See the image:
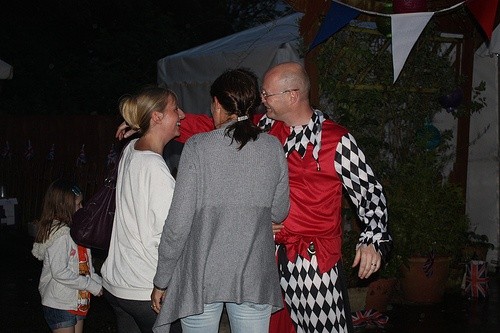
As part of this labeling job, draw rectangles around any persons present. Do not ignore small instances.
[28,180,103,333]
[150,69,291,333]
[114,61,388,333]
[97,85,185,332]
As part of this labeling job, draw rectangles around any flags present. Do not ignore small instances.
[348,307,388,330]
[459,260,489,300]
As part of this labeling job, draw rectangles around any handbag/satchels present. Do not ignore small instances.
[69,139,132,250]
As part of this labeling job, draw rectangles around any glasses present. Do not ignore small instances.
[71,184,82,197]
[260,86,300,100]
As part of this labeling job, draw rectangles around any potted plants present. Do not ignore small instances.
[399,166,472,306]
[456,231,495,261]
[365,188,411,311]
[342,229,370,312]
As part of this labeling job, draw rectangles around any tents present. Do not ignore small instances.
[157,12,307,119]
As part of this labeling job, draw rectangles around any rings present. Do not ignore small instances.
[371,263,375,266]
[152,305,155,307]
